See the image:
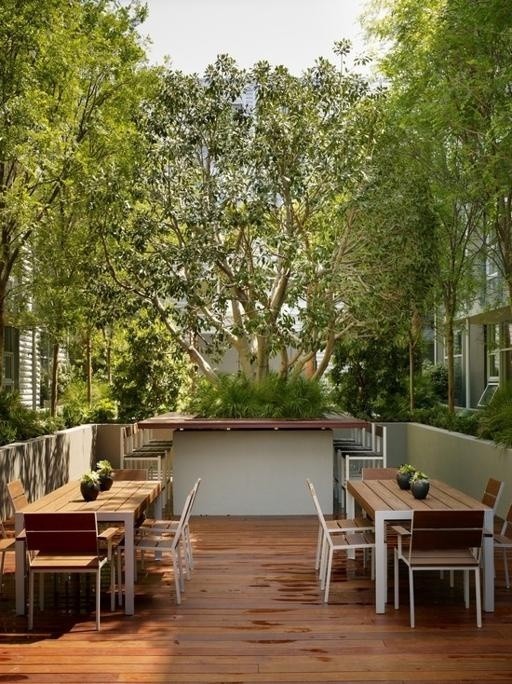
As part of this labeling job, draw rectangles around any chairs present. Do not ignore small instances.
[1,518,17,579]
[390,505,495,628]
[438,472,505,587]
[18,510,120,630]
[307,482,391,605]
[459,504,511,610]
[134,476,200,582]
[360,464,410,569]
[91,467,151,483]
[6,477,29,517]
[311,483,375,579]
[116,486,196,607]
[121,424,174,509]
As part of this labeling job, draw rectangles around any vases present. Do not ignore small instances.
[395,471,412,489]
[410,480,432,501]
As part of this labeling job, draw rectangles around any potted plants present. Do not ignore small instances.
[76,468,103,501]
[95,459,116,492]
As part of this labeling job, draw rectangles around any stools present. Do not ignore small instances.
[331,424,389,510]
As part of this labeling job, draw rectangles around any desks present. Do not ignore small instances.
[15,477,166,617]
[344,475,496,616]
